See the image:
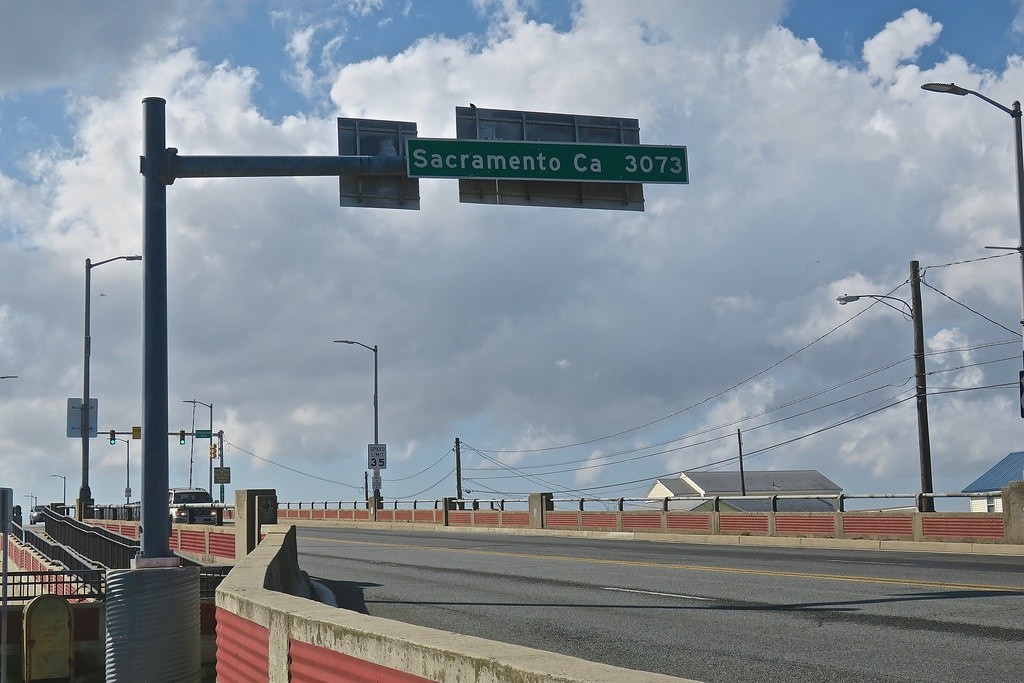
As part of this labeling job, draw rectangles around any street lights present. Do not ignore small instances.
[182,400,212,497]
[1,376,18,379]
[835,294,934,512]
[921,82,1024,418]
[79,254,142,499]
[24,495,37,506]
[333,339,378,444]
[107,437,129,503]
[49,474,66,505]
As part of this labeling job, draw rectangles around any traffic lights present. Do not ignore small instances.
[110,430,115,445]
[210,444,216,459]
[179,429,185,444]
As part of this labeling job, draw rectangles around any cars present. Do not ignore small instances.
[30,504,50,525]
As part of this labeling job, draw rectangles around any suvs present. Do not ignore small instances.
[169,487,219,525]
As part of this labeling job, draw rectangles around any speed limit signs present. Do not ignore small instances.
[368,444,386,469]
